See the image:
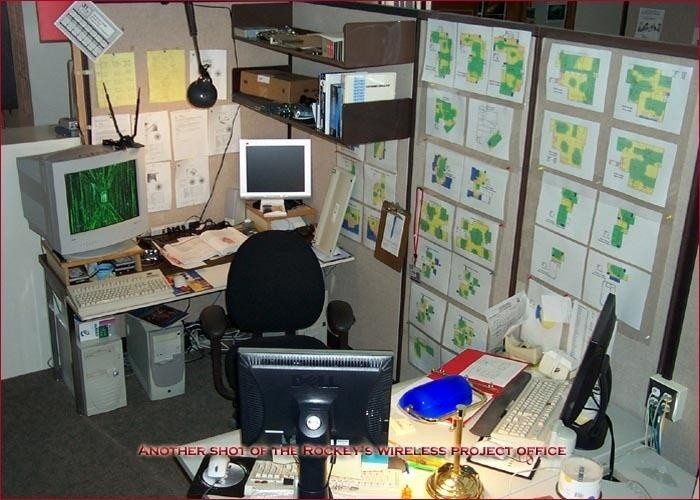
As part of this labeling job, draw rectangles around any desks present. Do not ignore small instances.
[503,443,699,499]
[33,223,356,404]
[174,340,659,500]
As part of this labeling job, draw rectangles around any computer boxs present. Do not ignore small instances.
[74,335,128,418]
[126,313,187,402]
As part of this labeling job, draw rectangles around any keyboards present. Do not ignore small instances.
[490,375,571,449]
[67,268,176,320]
[244,460,403,500]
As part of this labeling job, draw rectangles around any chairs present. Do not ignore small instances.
[191,230,353,408]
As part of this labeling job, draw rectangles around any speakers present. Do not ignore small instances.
[224,188,246,226]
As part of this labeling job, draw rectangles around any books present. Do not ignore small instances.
[163,227,249,265]
[72,313,115,343]
[317,34,397,140]
[126,304,189,329]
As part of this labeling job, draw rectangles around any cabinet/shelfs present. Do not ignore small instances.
[225,1,415,145]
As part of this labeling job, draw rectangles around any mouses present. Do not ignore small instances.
[207,454,231,479]
[480,439,509,460]
[173,274,186,287]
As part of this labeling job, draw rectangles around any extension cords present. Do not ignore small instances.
[187,335,211,350]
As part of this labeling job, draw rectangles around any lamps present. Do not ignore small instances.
[394,364,488,500]
[182,0,217,107]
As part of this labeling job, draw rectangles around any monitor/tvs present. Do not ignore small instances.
[14,143,151,260]
[234,346,395,500]
[560,292,618,452]
[238,138,313,218]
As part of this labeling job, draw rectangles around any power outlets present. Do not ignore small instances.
[645,372,687,423]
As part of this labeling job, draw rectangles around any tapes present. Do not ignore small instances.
[519,341,531,348]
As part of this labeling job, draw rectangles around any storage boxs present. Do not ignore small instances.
[239,67,318,104]
